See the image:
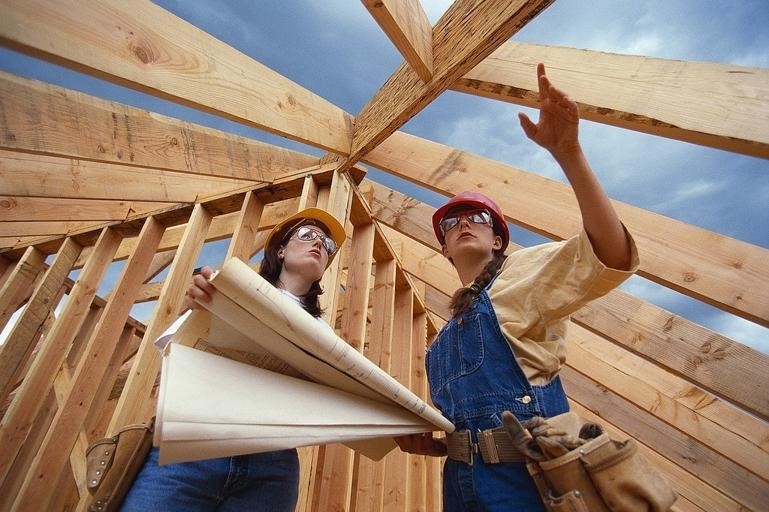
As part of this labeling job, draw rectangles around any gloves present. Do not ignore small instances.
[502,409,603,459]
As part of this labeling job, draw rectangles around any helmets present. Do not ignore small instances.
[433,192,509,253]
[266,208,347,269]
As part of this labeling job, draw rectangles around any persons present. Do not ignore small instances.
[392,63,641,512]
[119,207,347,512]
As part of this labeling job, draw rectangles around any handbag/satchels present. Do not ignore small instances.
[85,423,152,511]
[527,432,678,511]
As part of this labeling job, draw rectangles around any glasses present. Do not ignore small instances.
[440,210,492,230]
[290,227,337,255]
